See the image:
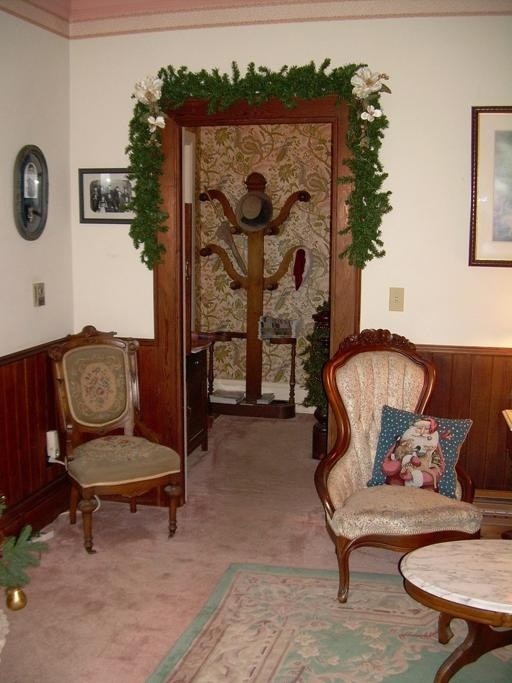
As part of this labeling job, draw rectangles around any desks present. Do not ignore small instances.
[391,535,510,682]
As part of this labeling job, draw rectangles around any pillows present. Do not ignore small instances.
[364,399,473,501]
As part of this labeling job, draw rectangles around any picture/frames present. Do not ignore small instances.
[76,166,139,225]
[465,103,511,267]
[14,143,52,242]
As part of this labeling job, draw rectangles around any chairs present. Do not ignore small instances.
[43,324,185,553]
[314,326,488,605]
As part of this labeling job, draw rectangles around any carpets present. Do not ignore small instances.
[141,560,509,682]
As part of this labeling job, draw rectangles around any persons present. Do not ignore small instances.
[27,164,37,197]
[89,181,132,211]
